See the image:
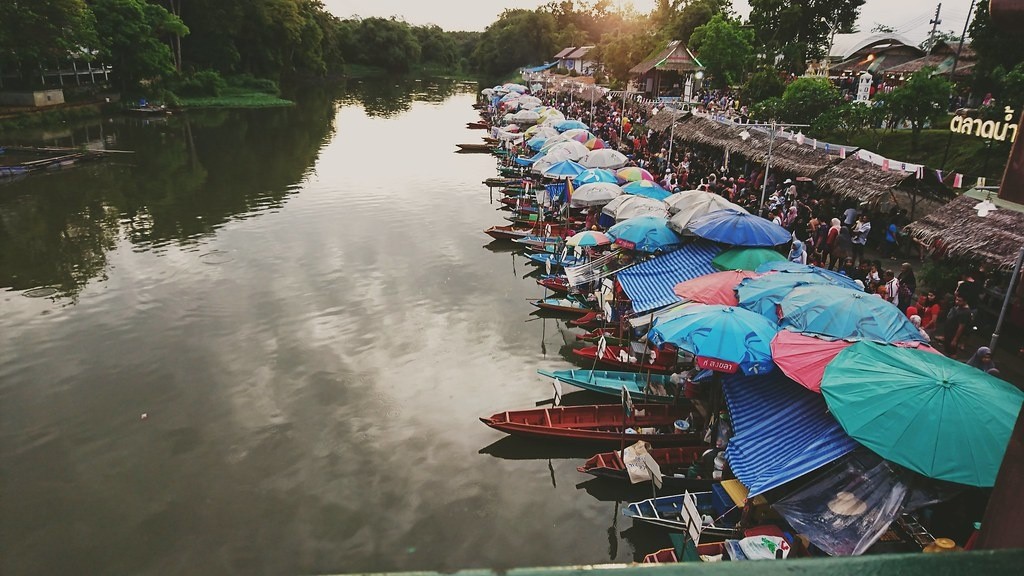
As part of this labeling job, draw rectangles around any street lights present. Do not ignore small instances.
[820,16,839,75]
[737,121,811,217]
[606,91,645,144]
[649,101,698,169]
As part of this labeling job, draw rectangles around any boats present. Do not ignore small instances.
[478,403,709,444]
[530,298,589,313]
[567,311,603,326]
[622,478,783,534]
[643,534,791,563]
[572,346,689,373]
[503,216,585,229]
[457,101,583,224]
[574,327,631,346]
[576,445,726,483]
[525,244,576,254]
[484,226,571,240]
[510,234,564,244]
[0,149,105,178]
[127,99,166,118]
[537,370,686,402]
[523,252,589,267]
[536,278,572,293]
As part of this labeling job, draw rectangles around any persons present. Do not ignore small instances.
[518,53,1008,375]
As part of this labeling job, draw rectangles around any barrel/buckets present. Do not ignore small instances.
[674,420,690,435]
[529,214,538,220]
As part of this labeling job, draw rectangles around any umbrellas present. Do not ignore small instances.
[481,83,1023,488]
